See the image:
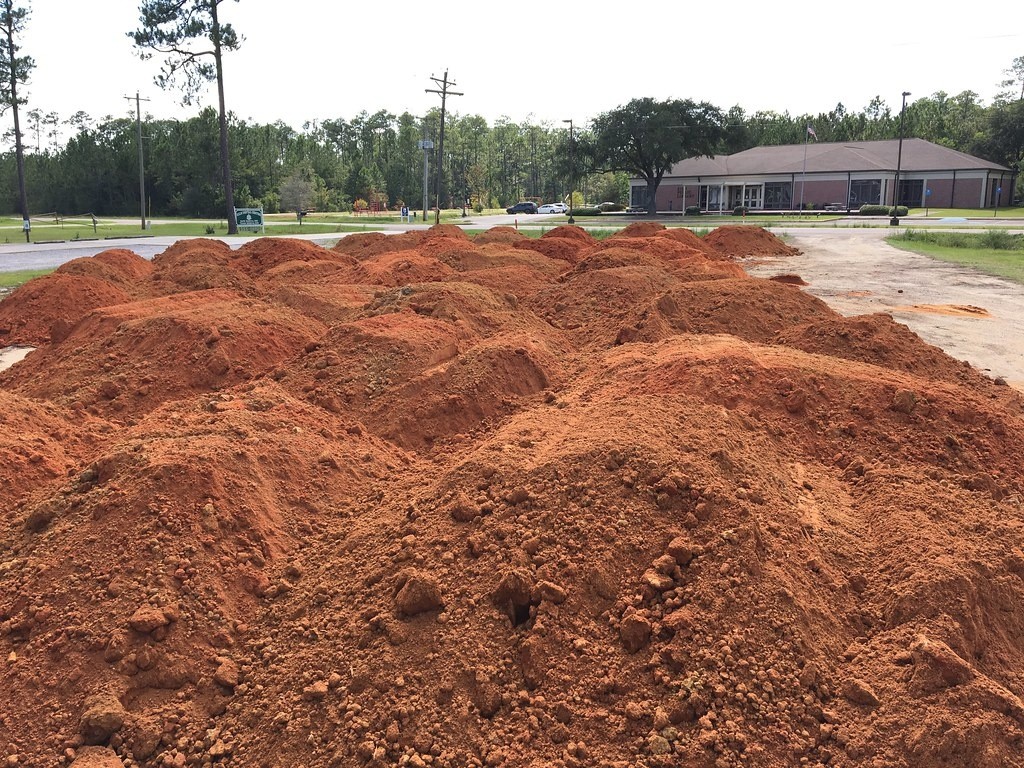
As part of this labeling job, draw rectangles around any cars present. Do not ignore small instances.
[553,202,569,213]
[536,203,563,214]
[594,201,615,212]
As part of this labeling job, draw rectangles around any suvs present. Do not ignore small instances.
[506,202,538,215]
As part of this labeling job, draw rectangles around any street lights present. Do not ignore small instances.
[890,91,912,226]
[562,118,575,224]
